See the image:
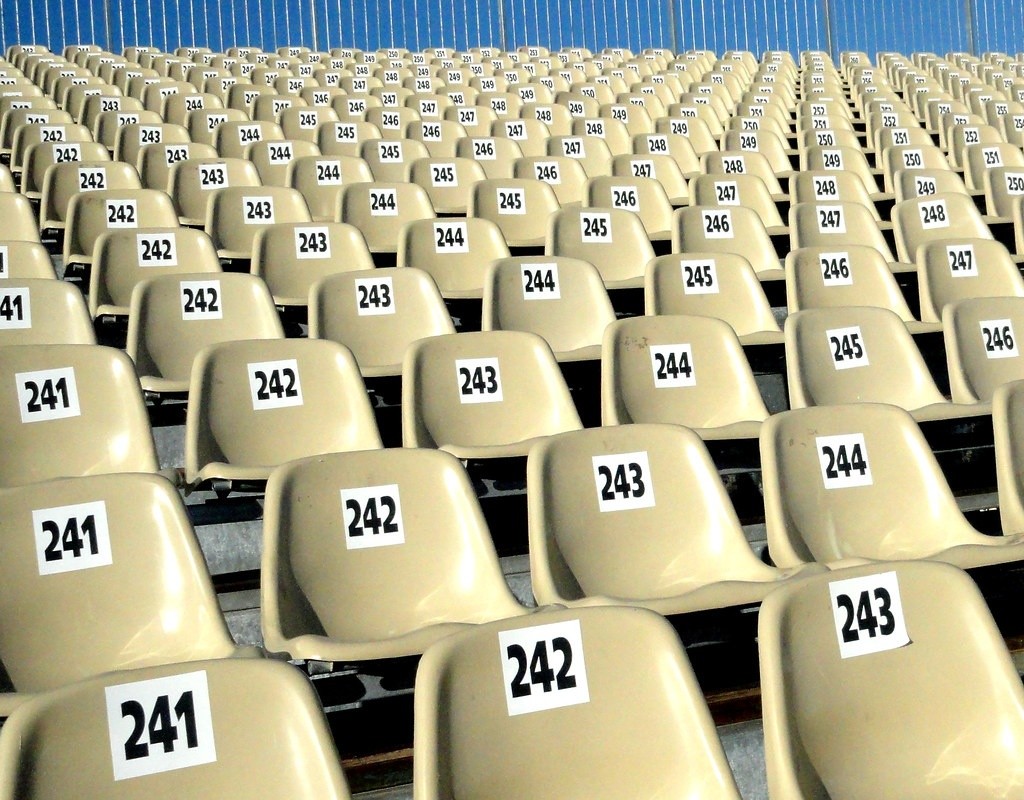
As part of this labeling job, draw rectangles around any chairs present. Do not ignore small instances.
[0,44,1024,800]
